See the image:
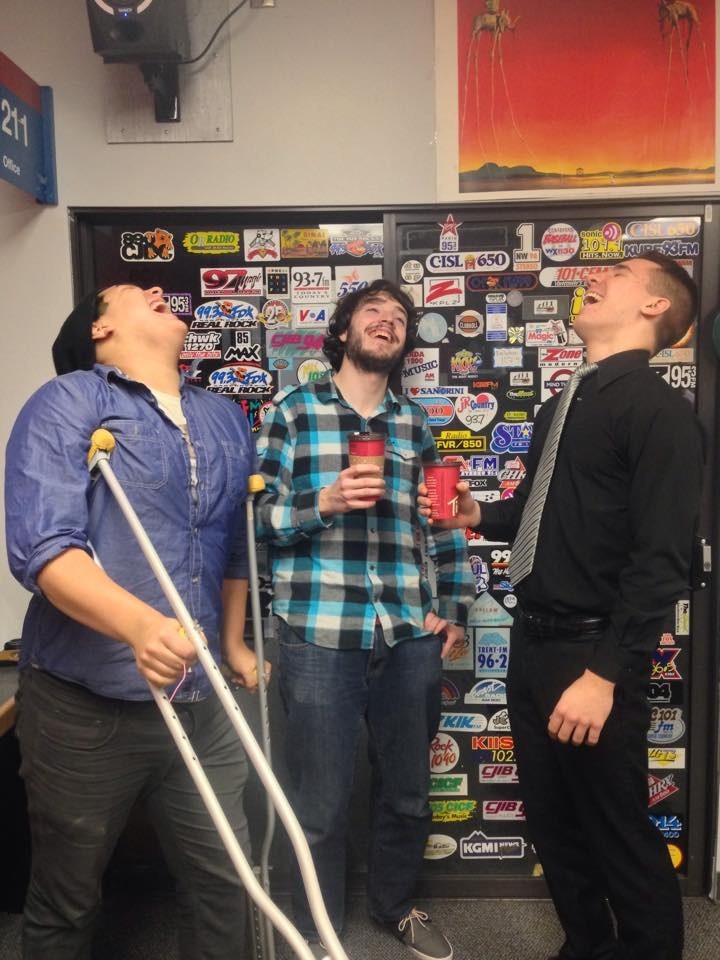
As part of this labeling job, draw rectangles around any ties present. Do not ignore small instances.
[509,360,600,585]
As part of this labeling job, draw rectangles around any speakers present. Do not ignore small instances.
[87,0,189,62]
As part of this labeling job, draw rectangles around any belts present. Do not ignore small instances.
[518,610,608,633]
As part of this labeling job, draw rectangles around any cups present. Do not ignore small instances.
[346,431,388,501]
[421,462,460,520]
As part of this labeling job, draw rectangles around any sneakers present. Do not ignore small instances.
[374,905,453,960]
[292,934,333,960]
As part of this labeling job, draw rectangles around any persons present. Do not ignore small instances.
[418,249,712,960]
[253,280,469,960]
[5,283,273,960]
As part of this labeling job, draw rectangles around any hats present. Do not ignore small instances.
[52,290,97,374]
